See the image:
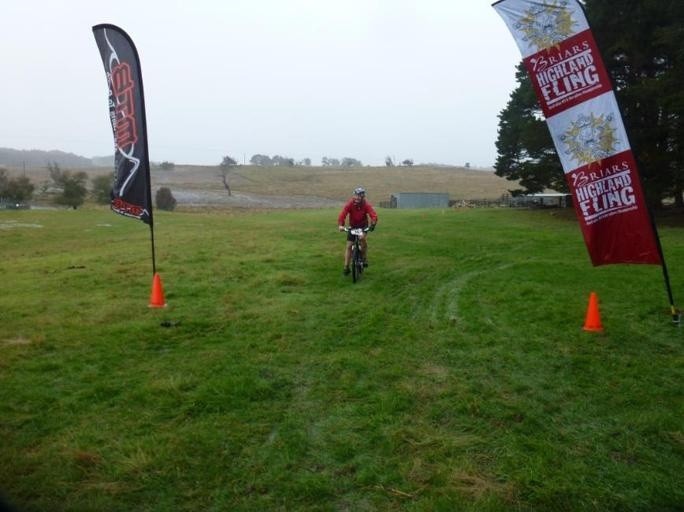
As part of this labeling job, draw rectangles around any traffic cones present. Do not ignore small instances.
[582,291,603,333]
[148,272,165,309]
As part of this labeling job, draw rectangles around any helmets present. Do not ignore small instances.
[353,187,366,196]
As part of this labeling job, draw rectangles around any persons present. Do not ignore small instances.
[338,187,377,276]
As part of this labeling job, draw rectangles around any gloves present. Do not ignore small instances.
[369,224,376,232]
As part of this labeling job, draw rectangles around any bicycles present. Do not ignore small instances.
[342,226,368,284]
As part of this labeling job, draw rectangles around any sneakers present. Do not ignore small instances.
[363,258,368,264]
[343,265,349,272]
